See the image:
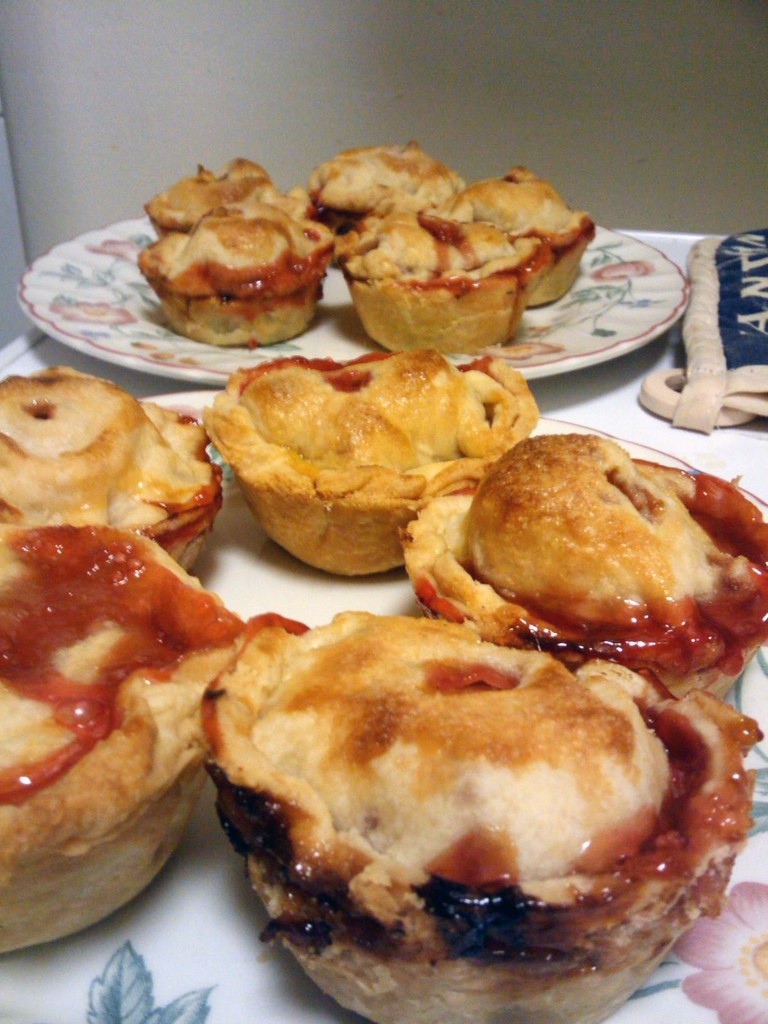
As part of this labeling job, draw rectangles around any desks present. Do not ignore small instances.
[0,228,768,1024]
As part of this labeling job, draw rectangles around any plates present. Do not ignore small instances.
[0,392,768,1023]
[19,214,687,387]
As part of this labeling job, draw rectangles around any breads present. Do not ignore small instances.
[0,144,768,1024]
